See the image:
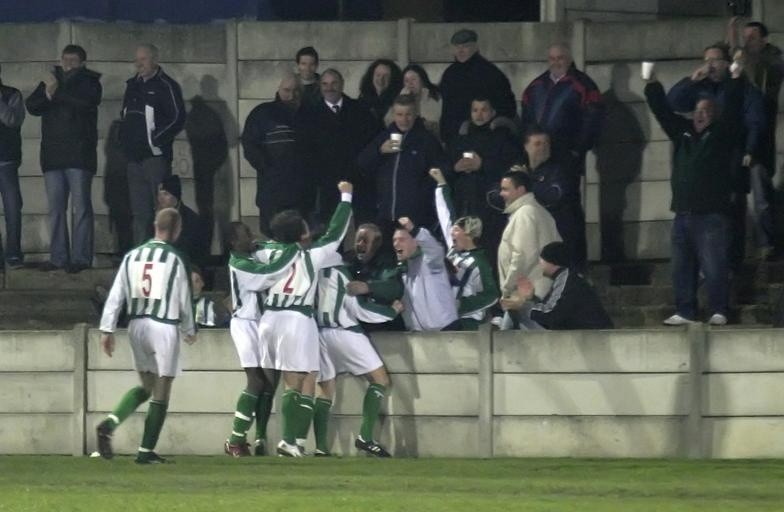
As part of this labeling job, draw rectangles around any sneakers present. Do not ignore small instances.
[135,452,164,463]
[97,424,111,456]
[356,434,390,456]
[709,314,727,325]
[664,314,702,325]
[225,438,327,457]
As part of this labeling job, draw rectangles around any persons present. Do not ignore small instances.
[26,45,102,273]
[0,78,25,276]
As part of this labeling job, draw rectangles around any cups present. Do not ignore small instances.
[729,60,742,71]
[389,133,401,151]
[463,152,471,158]
[641,62,654,80]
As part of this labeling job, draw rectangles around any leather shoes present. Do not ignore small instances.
[40,261,87,272]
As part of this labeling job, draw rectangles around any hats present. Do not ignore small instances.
[451,30,478,44]
[541,241,571,266]
[158,175,181,200]
